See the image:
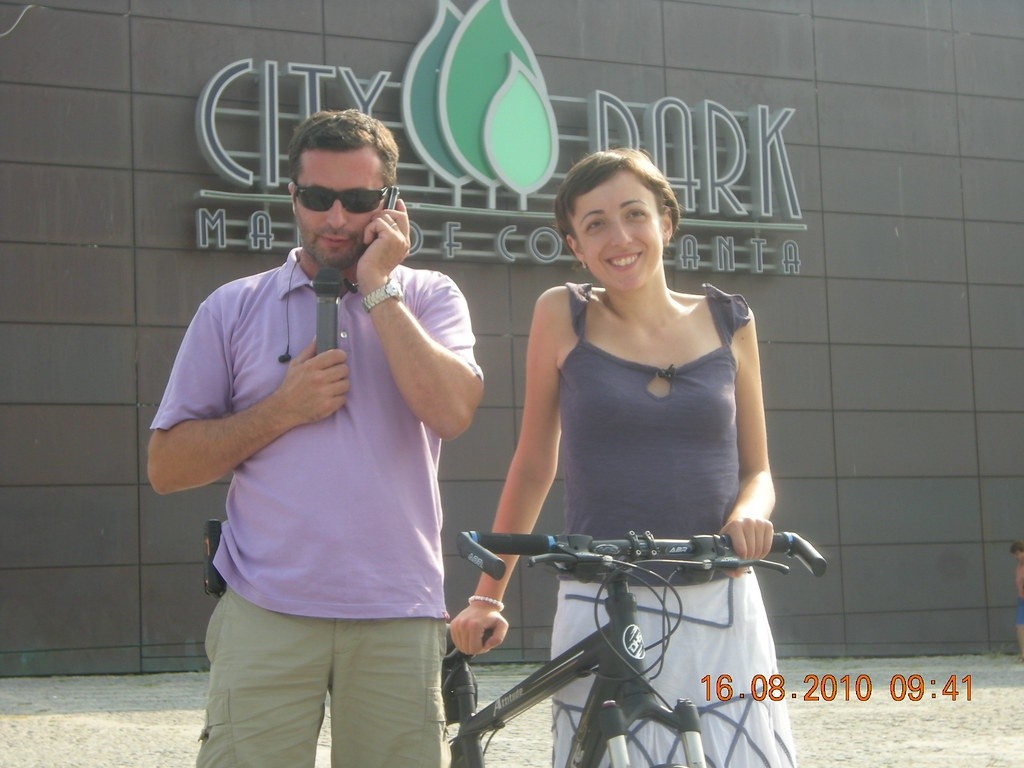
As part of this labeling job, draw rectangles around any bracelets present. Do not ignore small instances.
[390,222,398,227]
[467,594,505,613]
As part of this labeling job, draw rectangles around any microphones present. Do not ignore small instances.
[312,267,344,356]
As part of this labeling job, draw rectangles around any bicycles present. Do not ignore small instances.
[201,519,828,768]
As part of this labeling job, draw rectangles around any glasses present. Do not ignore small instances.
[294,182,388,213]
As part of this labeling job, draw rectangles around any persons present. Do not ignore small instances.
[145,106,486,768]
[448,144,800,768]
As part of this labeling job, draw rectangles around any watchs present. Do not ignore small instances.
[360,276,404,314]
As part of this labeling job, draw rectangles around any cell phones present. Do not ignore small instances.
[387,186,400,211]
